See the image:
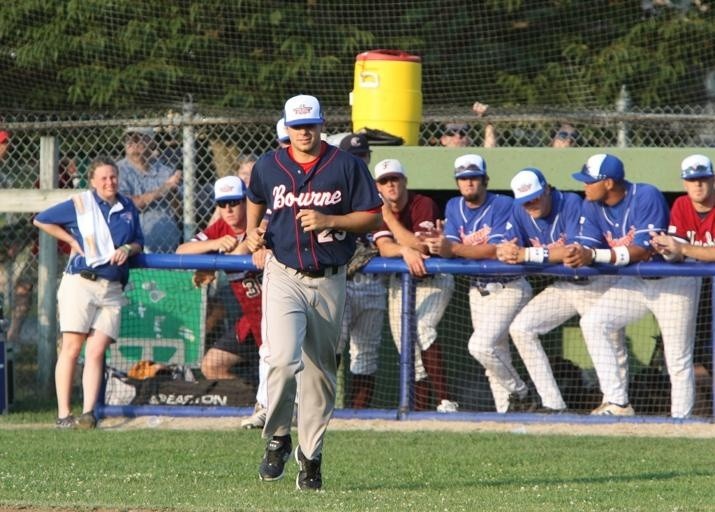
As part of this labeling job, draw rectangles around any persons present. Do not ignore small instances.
[246,93,384,493]
[1,123,303,430]
[319,122,715,415]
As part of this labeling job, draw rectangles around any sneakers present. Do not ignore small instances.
[294,443,322,493]
[240,408,270,430]
[79,410,99,432]
[435,398,459,413]
[258,435,293,482]
[505,389,539,414]
[54,416,78,431]
[589,402,636,416]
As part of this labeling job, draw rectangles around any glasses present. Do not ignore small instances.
[555,130,582,141]
[442,127,471,137]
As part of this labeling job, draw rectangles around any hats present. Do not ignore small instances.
[283,94,323,127]
[374,158,406,182]
[510,167,547,206]
[454,153,487,179]
[214,174,247,202]
[572,153,625,184]
[119,120,154,147]
[680,153,714,180]
[276,117,290,142]
[339,133,373,154]
